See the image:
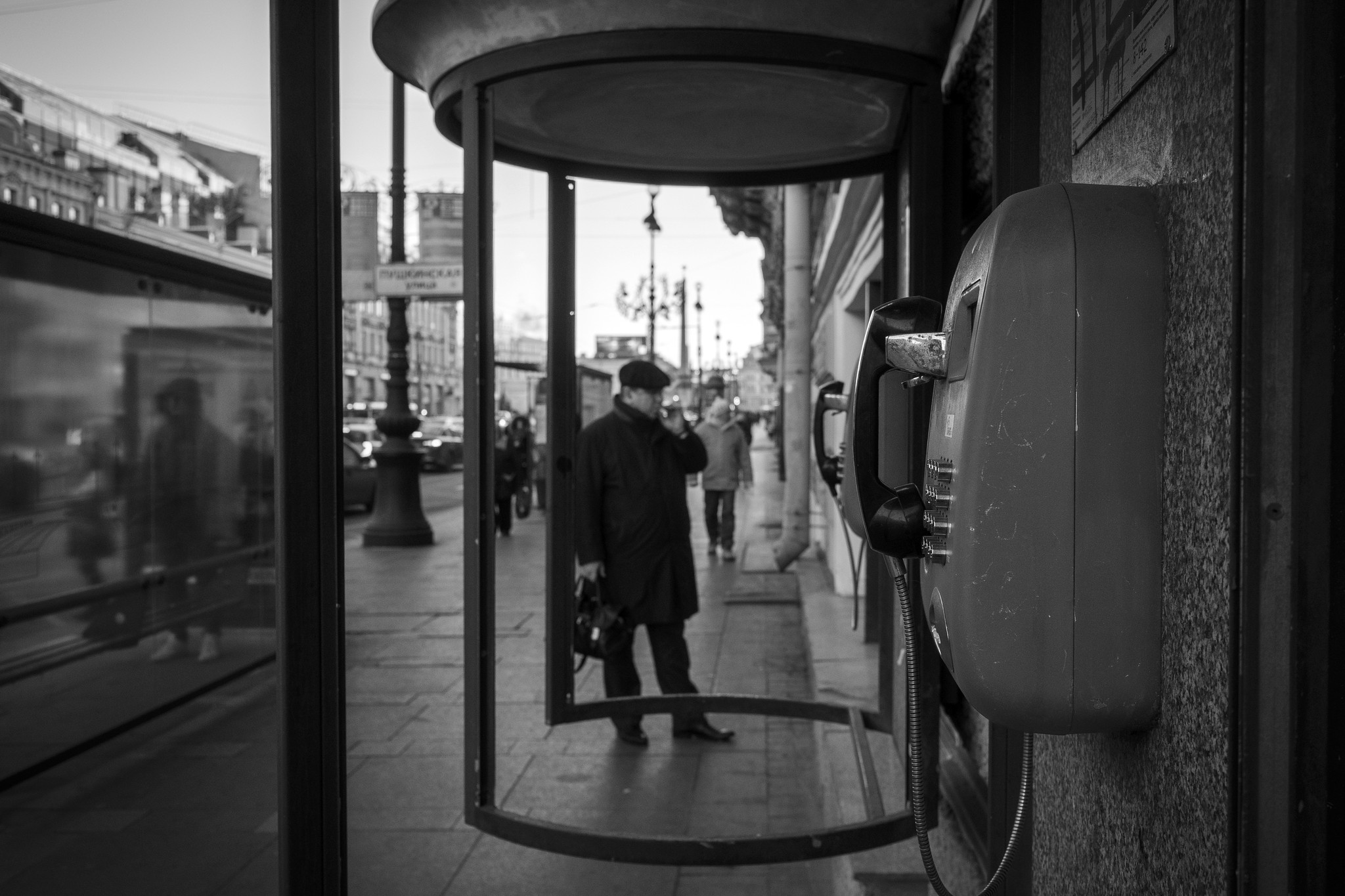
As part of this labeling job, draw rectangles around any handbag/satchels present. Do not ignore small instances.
[574,576,624,673]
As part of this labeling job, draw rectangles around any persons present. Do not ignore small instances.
[150,616,226,665]
[490,408,544,544]
[685,397,755,562]
[572,361,739,742]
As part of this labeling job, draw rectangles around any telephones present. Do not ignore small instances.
[813,380,845,486]
[842,184,1168,736]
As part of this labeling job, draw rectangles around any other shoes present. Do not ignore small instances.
[709,541,717,555]
[723,549,735,561]
[148,634,188,663]
[199,632,222,663]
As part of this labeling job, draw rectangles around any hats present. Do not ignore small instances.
[620,360,670,388]
[711,396,731,416]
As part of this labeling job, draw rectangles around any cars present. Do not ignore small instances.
[161,390,500,547]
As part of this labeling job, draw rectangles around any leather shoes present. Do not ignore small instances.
[672,717,735,741]
[616,725,649,747]
